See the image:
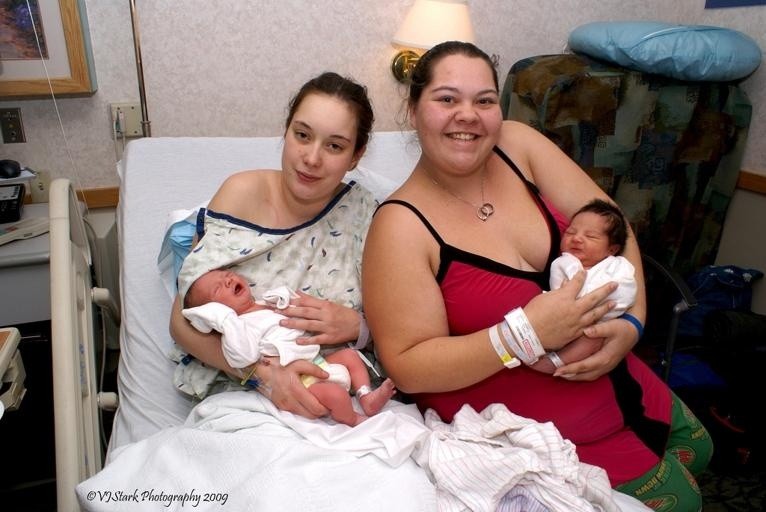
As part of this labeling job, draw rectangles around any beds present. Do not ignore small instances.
[48,130,653,512]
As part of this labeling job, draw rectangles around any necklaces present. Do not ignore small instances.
[418,154,496,221]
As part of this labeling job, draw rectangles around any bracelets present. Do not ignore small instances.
[619,311,644,339]
[347,311,372,351]
[489,307,544,370]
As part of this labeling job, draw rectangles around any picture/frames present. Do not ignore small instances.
[0,0,98,101]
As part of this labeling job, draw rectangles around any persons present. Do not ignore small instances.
[169,72,385,403]
[528,199,638,374]
[184,268,397,427]
[361,42,714,512]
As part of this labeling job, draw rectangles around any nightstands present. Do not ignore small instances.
[0,201,85,512]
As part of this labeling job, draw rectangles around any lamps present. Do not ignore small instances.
[391,0,474,83]
